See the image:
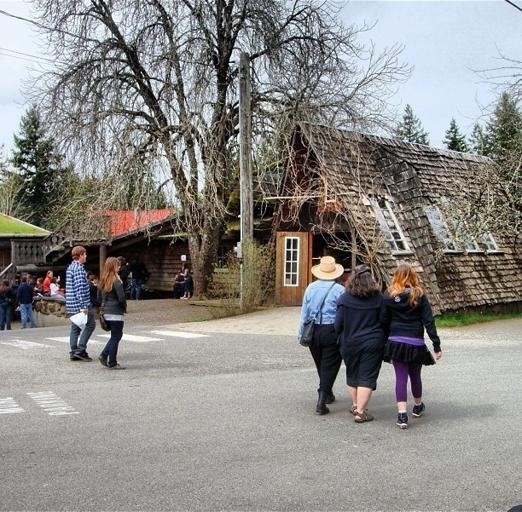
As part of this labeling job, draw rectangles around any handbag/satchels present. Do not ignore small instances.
[300,320,315,346]
[99,314,111,331]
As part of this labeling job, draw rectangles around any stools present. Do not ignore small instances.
[27,291,66,316]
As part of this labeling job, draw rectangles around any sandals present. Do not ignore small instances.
[349,406,373,423]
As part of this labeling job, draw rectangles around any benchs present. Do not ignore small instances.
[144,268,182,292]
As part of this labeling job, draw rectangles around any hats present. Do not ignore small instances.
[311,256,345,280]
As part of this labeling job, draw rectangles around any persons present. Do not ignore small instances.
[294,255,346,416]
[95,256,129,371]
[174,262,192,302]
[378,261,441,429]
[65,247,97,364]
[1,256,142,332]
[334,263,385,423]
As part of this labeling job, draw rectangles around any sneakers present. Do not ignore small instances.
[109,364,126,370]
[98,355,108,367]
[396,413,408,429]
[412,403,426,417]
[70,352,91,362]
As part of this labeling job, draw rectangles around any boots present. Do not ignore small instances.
[316,391,335,415]
